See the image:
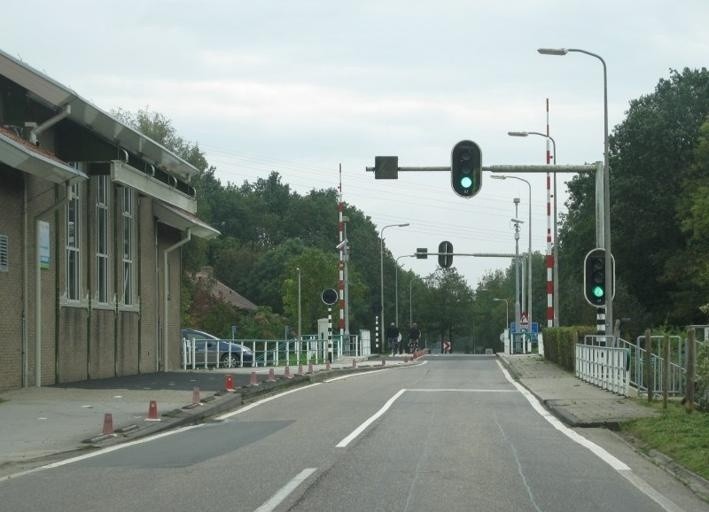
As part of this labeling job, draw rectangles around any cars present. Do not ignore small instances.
[182,328,254,369]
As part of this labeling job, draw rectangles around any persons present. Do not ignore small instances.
[408,323,422,353]
[387,321,399,353]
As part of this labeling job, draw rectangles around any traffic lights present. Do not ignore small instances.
[451,140,482,198]
[584,248,616,307]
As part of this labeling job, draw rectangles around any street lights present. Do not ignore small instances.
[491,131,560,332]
[296,265,301,335]
[537,47,613,346]
[381,223,429,355]
